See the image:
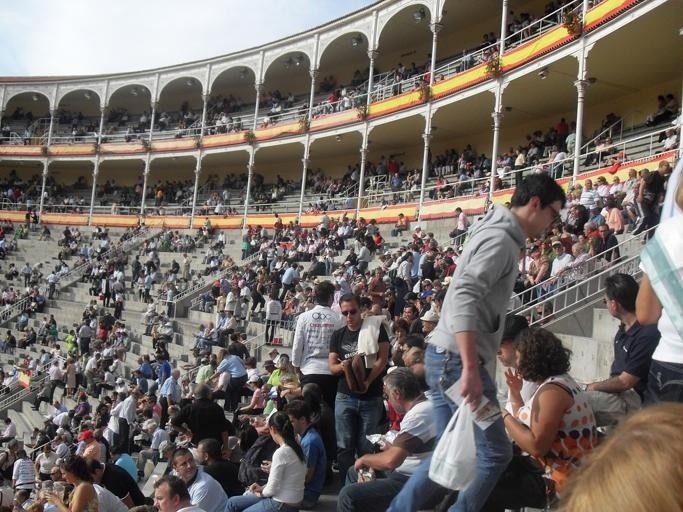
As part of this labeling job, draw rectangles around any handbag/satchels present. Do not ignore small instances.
[238,435,276,486]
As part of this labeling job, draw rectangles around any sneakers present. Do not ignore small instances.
[31,406,39,411]
[138,471,144,477]
[88,392,99,398]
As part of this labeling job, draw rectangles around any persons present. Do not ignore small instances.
[1,1,683,511]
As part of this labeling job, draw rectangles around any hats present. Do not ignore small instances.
[414,226,420,231]
[80,431,92,439]
[116,378,123,384]
[159,440,173,458]
[420,311,439,322]
[502,314,528,340]
[246,375,259,385]
[56,428,64,434]
[263,359,276,366]
[552,241,562,247]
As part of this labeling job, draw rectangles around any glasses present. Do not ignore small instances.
[342,309,359,316]
[547,205,562,229]
